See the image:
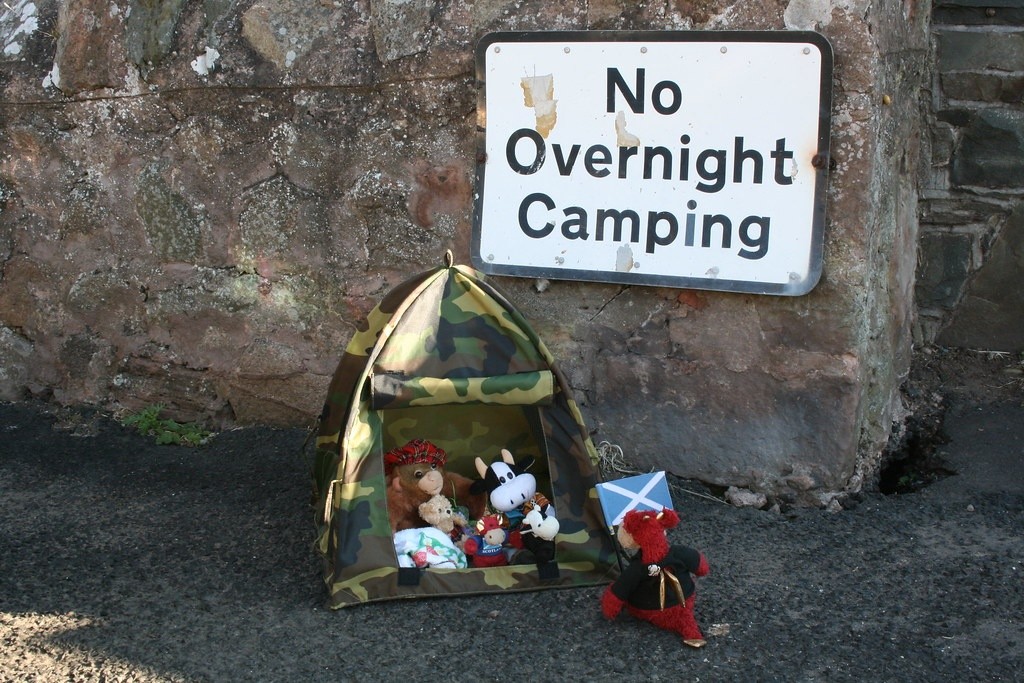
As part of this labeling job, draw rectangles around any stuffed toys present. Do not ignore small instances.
[602,509,708,641]
[383,437,560,569]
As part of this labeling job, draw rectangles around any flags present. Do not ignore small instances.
[596,472,674,526]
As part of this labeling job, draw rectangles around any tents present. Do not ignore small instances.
[312,249,622,611]
